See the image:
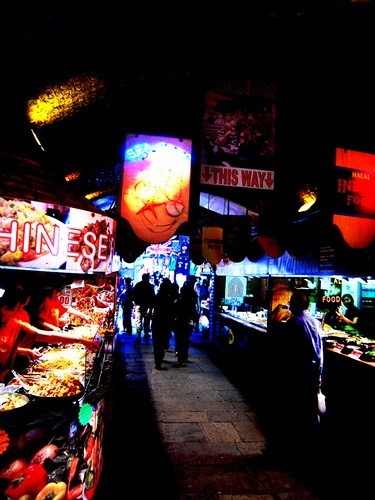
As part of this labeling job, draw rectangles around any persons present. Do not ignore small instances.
[0,275,102,371]
[258,288,324,473]
[174,285,201,367]
[333,294,361,336]
[122,272,209,338]
[148,283,175,370]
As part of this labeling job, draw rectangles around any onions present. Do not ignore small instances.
[0,444,58,481]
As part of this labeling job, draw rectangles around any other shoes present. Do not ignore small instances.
[144,334,151,339]
[155,365,168,370]
[175,361,187,368]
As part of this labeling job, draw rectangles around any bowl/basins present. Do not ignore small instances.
[0,378,85,423]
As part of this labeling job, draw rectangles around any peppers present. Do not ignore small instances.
[5,465,67,500]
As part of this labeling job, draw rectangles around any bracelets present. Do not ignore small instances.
[76,337,80,343]
